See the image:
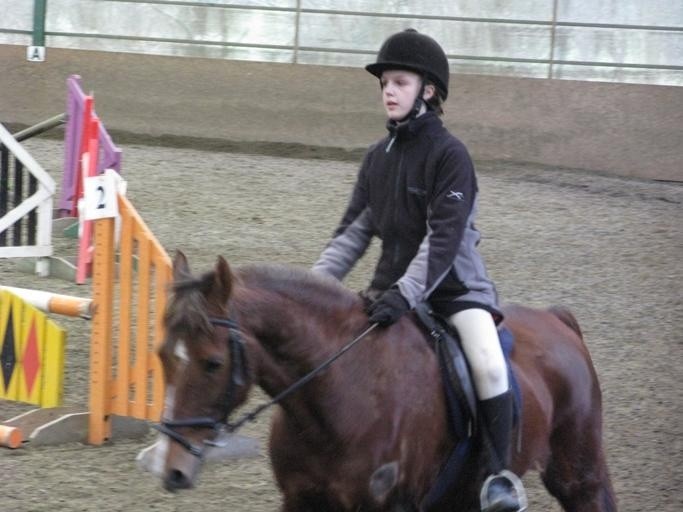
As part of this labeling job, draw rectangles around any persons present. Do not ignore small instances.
[309,28,521,512]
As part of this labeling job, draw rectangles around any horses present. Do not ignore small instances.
[147,247,622,512]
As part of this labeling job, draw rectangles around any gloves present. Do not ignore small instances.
[366,288,410,329]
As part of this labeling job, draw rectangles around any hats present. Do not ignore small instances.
[364,27,450,102]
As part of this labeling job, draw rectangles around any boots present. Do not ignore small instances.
[478,384,518,511]
